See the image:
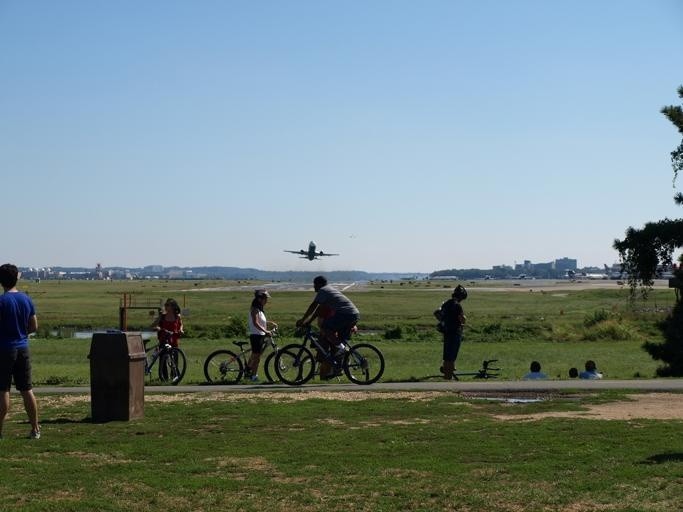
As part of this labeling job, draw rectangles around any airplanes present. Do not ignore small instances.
[283,241,340,260]
[511,273,533,280]
[565,270,609,281]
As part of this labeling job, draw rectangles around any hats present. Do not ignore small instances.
[255,289,271,298]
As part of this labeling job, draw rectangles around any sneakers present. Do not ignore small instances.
[30,428,41,440]
[334,343,346,357]
[243,369,259,382]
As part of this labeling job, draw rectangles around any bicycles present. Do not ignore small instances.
[202,322,303,386]
[274,320,385,385]
[422,357,500,381]
[141,328,187,385]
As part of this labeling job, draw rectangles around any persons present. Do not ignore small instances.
[0,268,39,438]
[316,305,357,381]
[433,284,467,379]
[245,289,278,381]
[579,360,601,378]
[568,367,579,378]
[522,361,547,380]
[296,275,360,378]
[150,297,182,382]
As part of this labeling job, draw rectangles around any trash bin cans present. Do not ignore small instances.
[87,329,146,422]
